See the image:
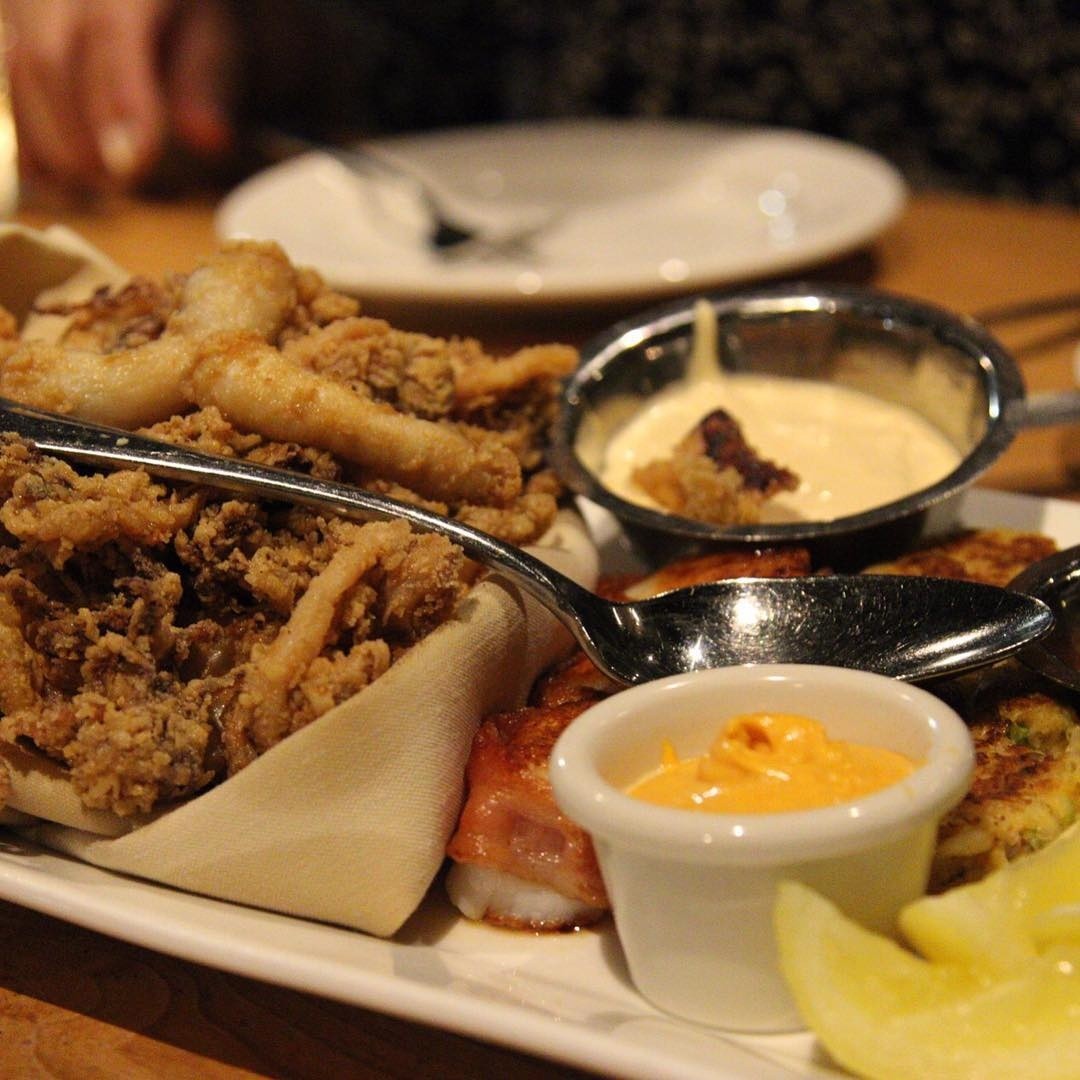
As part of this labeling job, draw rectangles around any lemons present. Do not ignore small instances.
[773,808,1080,1079]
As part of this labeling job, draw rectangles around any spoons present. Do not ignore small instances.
[1,399,1053,683]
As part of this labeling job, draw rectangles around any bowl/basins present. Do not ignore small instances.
[548,288,1029,574]
[547,662,974,1035]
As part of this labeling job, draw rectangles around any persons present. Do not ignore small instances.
[10,3,1080,216]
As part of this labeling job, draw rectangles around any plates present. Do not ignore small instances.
[214,124,914,329]
[1,484,1080,1080]
